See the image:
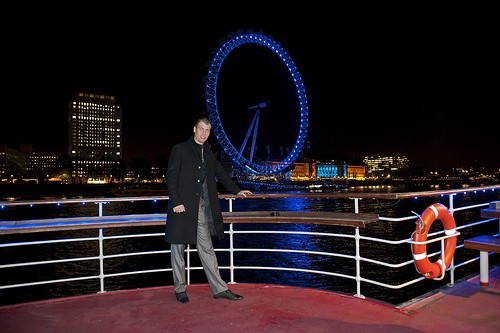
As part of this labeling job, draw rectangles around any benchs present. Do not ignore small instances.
[464,235,500,286]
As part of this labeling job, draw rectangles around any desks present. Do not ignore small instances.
[481,209,500,218]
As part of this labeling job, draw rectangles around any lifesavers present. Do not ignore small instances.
[411,203,457,277]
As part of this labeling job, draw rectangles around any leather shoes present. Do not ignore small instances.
[213,288,243,299]
[175,291,189,303]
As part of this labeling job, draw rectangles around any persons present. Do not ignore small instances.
[165,118,254,304]
